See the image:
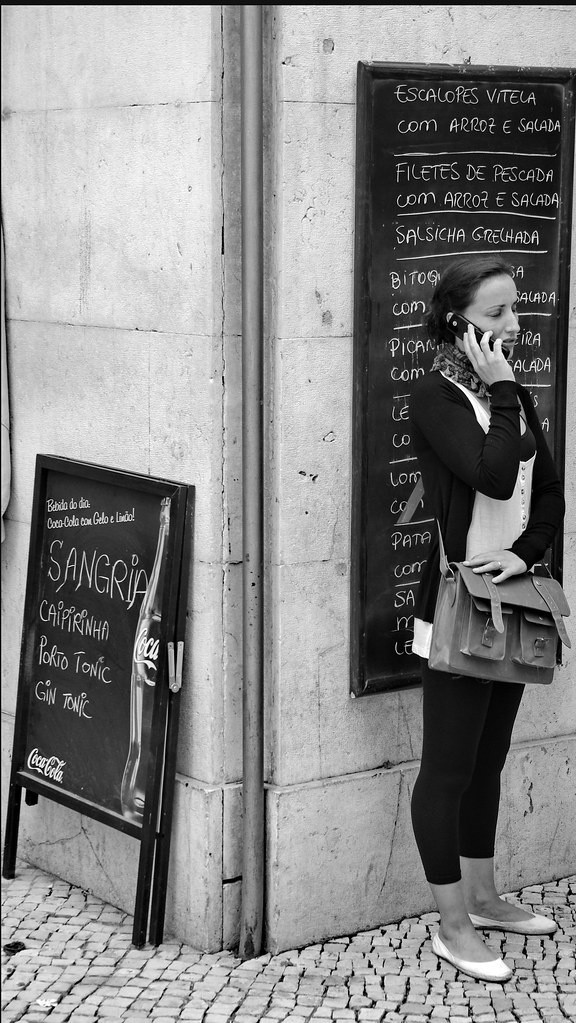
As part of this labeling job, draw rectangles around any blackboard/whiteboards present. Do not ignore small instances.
[14,454,196,841]
[349,60,576,697]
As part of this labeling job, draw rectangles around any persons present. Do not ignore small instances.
[406,257,567,982]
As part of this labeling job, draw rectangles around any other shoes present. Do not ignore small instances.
[431,932,512,981]
[468,904,557,934]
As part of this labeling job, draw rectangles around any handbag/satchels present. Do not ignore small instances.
[428,559,572,684]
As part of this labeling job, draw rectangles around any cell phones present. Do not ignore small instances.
[448,312,510,361]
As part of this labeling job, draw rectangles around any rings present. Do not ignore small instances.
[498,562,502,568]
